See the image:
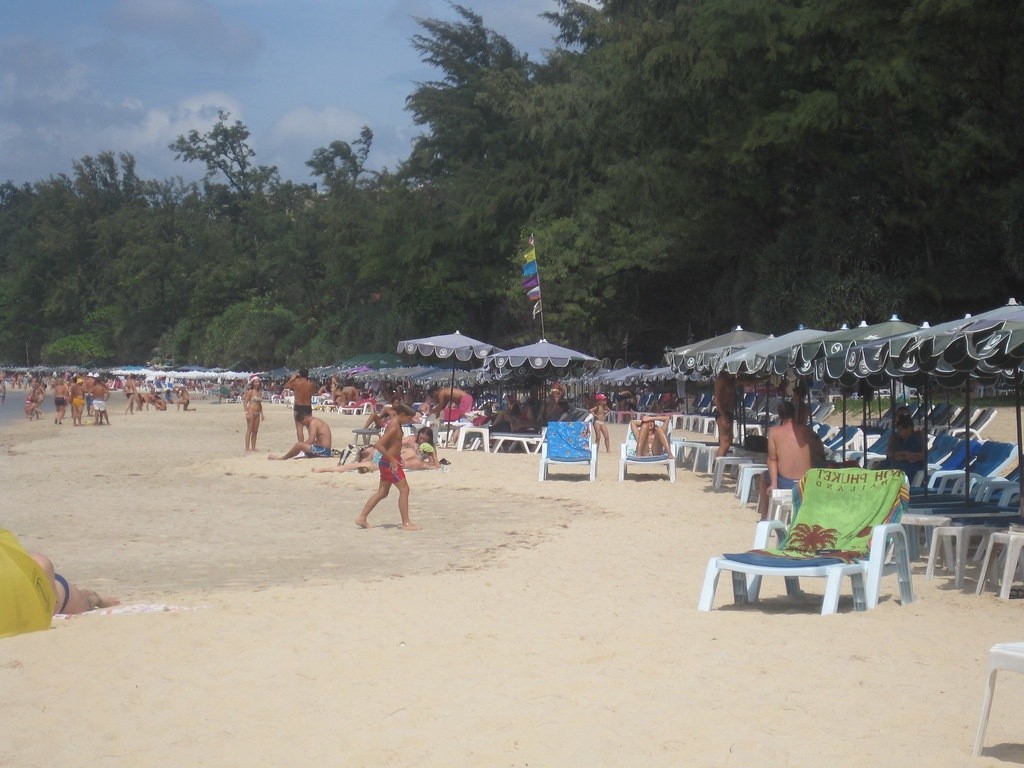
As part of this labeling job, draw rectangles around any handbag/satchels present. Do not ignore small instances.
[340,444,361,466]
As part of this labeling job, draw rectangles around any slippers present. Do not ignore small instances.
[440,458,451,465]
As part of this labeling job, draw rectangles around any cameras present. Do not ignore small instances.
[296,375,299,378]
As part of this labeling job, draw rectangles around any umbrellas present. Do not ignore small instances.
[0,297,1024,498]
[0,528,57,638]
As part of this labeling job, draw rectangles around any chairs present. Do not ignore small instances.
[130,375,1024,757]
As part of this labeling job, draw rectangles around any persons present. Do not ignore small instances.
[30,553,121,614]
[792,388,809,424]
[895,407,909,419]
[874,416,923,483]
[810,428,826,464]
[0,368,675,532]
[715,365,737,457]
[759,402,816,520]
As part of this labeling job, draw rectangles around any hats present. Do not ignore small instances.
[596,393,608,401]
[419,443,434,457]
